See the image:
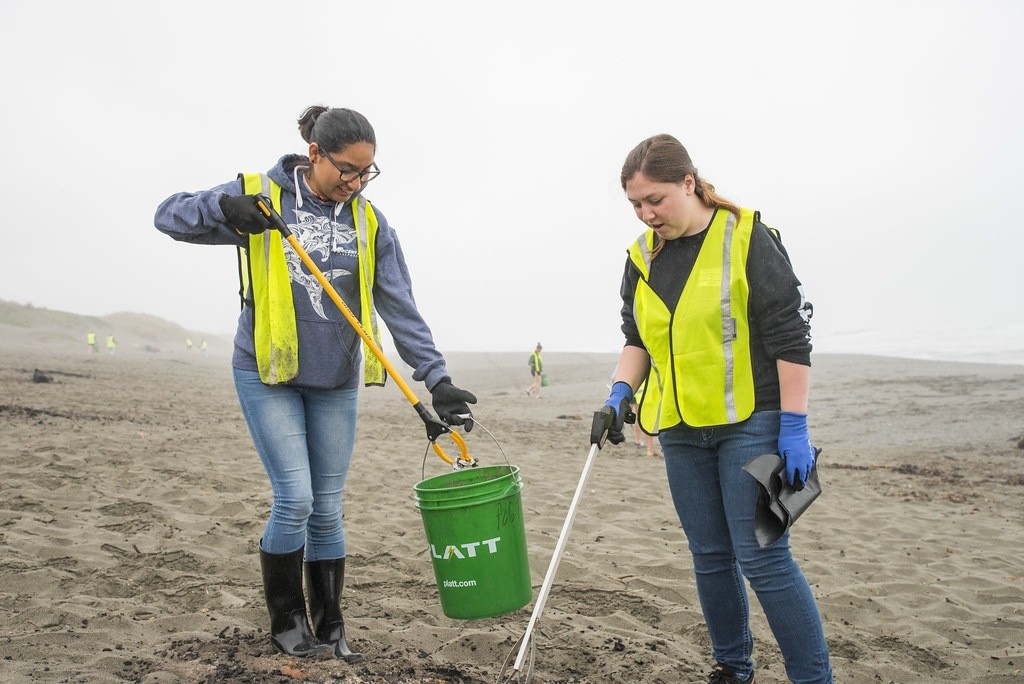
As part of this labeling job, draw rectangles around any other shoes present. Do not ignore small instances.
[707,663,755,684]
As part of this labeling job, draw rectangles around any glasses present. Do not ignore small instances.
[320,144,380,182]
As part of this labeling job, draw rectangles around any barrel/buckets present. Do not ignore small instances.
[413,413,532,620]
[541,374,549,387]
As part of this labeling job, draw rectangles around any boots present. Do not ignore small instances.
[303,557,363,664]
[259,537,329,658]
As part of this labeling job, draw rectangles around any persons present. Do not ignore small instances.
[602,135,834,684]
[185,337,192,351]
[527,341,544,399]
[107,333,118,354]
[154,102,476,664]
[86,329,96,355]
[198,338,207,349]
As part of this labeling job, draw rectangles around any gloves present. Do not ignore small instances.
[219,193,274,235]
[778,412,816,492]
[599,381,634,445]
[430,376,477,432]
